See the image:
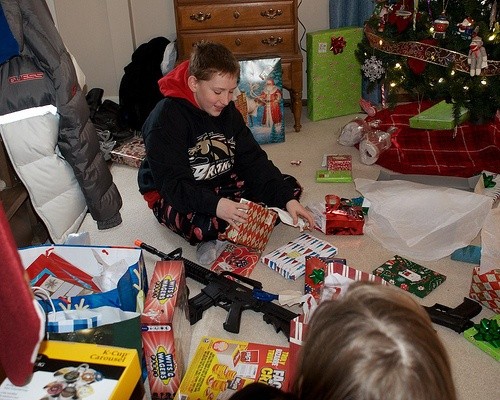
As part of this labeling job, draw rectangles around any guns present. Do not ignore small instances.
[420,297,483,334]
[134,240,301,342]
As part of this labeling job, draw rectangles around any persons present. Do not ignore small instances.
[137,42,314,245]
[229,279,458,400]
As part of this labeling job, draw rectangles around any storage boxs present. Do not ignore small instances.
[373,255,447,298]
[307,26,363,121]
[234,55,285,144]
[173,336,289,400]
[303,203,365,236]
[263,233,338,279]
[108,133,147,167]
[0,340,149,400]
[315,170,353,182]
[470,267,500,314]
[140,261,192,400]
[327,155,352,170]
[228,199,278,252]
[411,100,468,129]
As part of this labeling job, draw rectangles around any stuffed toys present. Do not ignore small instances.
[467,35,488,77]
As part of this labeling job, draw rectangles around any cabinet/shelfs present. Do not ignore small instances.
[174,0,303,133]
